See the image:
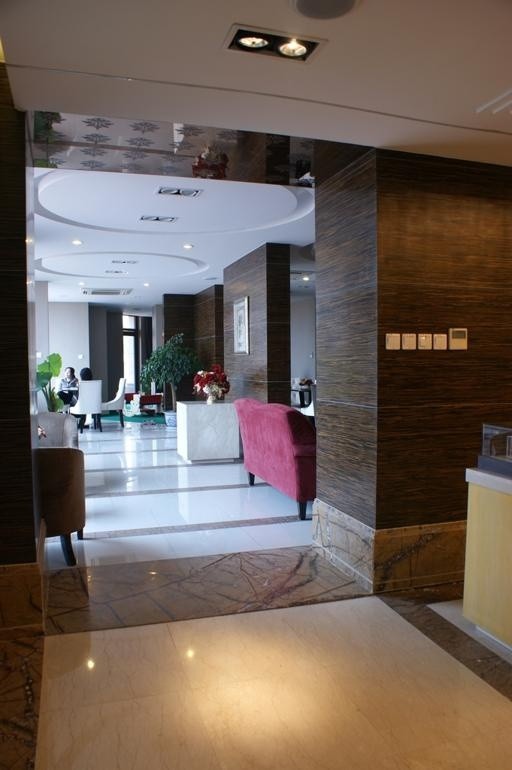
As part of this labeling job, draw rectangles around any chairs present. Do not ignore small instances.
[35,410,87,567]
[70,375,128,433]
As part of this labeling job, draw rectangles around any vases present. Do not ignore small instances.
[206,395,216,404]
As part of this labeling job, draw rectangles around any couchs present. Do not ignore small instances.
[234,396,315,521]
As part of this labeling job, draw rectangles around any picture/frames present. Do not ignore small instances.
[232,295,250,356]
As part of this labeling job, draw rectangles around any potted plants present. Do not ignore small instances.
[137,331,206,427]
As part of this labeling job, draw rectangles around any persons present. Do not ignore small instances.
[59,367,78,398]
[56,367,93,408]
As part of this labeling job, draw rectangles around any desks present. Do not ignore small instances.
[291,387,311,406]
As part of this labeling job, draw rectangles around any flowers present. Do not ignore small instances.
[191,362,231,400]
